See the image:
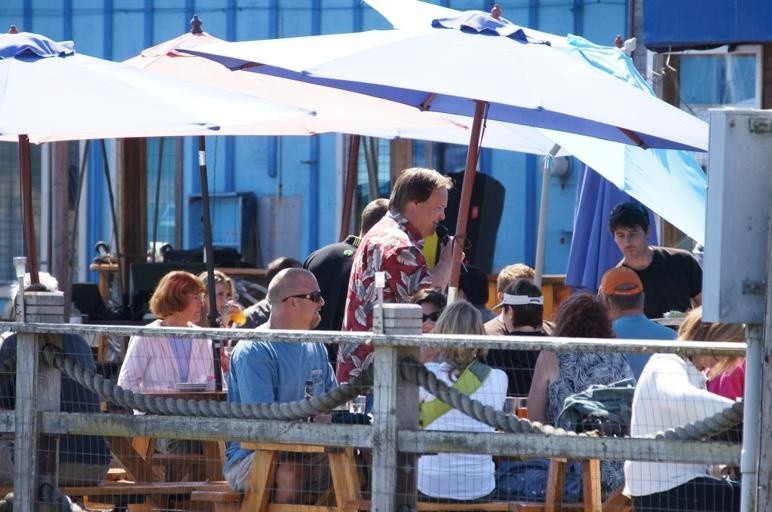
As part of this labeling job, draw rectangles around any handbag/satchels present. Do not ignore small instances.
[555,378,635,435]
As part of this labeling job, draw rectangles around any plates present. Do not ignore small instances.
[175,382,207,394]
[650,317,685,329]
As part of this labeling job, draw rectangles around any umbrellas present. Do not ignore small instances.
[0,22,319,285]
[173,6,718,286]
[110,18,473,391]
[377,105,564,166]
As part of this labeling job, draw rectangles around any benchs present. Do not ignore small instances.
[1,475,238,511]
[188,484,243,511]
[340,491,641,511]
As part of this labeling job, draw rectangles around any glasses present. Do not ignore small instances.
[422,312,440,322]
[281,291,323,303]
[610,202,646,215]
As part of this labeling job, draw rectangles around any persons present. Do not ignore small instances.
[238,254,306,327]
[299,196,393,379]
[194,270,242,328]
[405,286,449,361]
[595,198,706,321]
[337,166,468,413]
[404,298,512,505]
[455,265,501,321]
[226,264,352,508]
[1,284,112,505]
[480,277,565,400]
[624,304,752,511]
[591,268,687,389]
[114,267,228,485]
[476,258,561,340]
[521,291,640,494]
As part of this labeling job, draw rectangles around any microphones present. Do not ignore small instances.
[436,225,469,277]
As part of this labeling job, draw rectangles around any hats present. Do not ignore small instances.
[598,266,644,297]
[493,292,544,310]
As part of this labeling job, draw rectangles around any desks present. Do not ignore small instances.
[236,436,373,512]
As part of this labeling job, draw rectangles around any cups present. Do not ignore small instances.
[348,395,367,415]
[516,397,529,419]
[503,396,517,417]
[222,298,246,327]
